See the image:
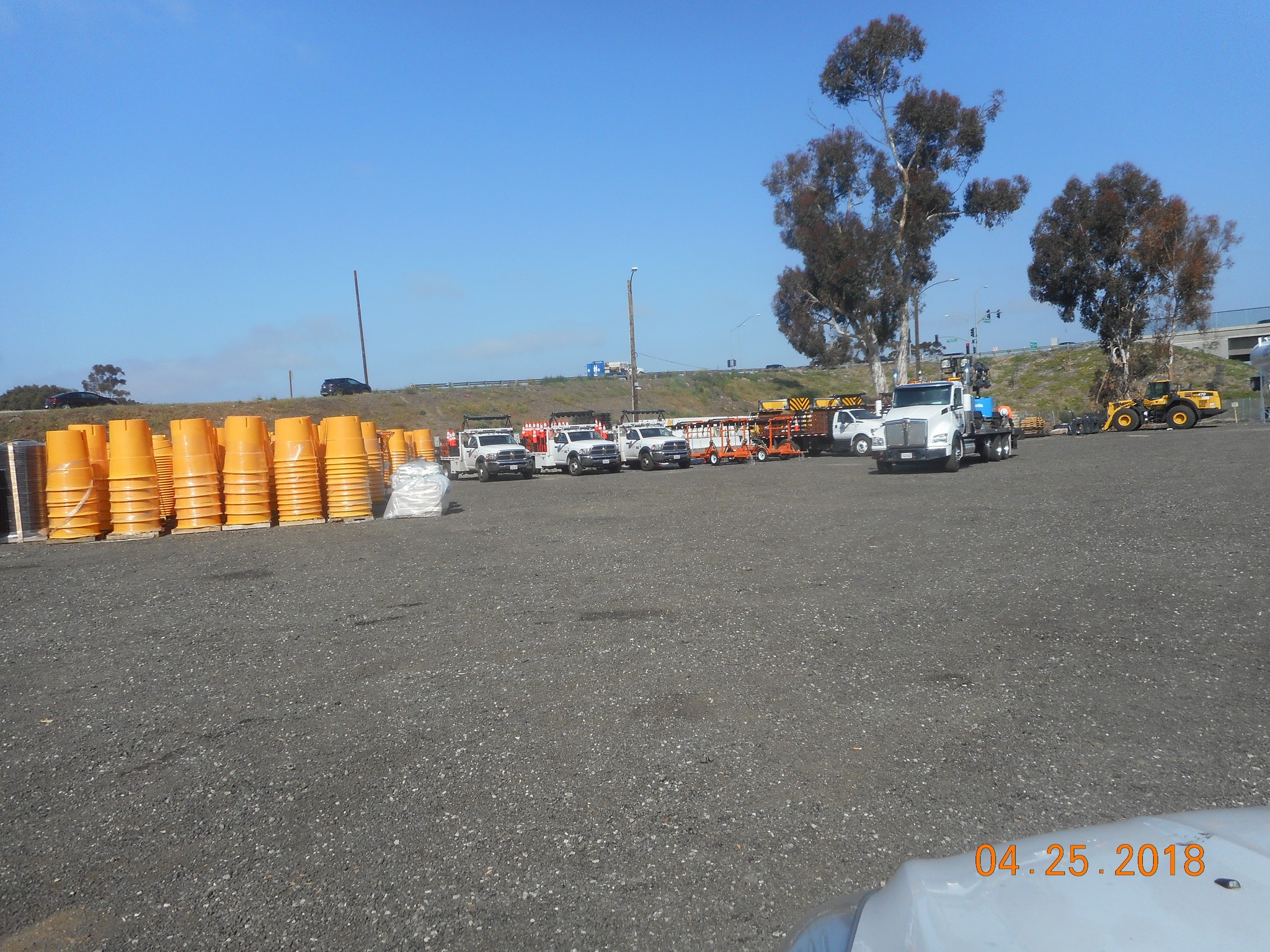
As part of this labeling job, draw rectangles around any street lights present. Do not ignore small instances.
[944,314,972,354]
[975,285,989,358]
[738,313,761,370]
[627,267,640,423]
[914,277,961,380]
[730,323,746,371]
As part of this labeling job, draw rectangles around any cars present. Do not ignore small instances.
[42,391,120,410]
[765,364,786,369]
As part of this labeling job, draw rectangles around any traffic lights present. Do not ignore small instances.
[996,309,1000,318]
[935,334,939,342]
[966,343,970,354]
[727,360,731,368]
[986,309,991,319]
[733,359,737,367]
[970,327,975,337]
[973,346,976,354]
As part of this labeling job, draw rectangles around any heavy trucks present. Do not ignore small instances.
[871,355,1024,473]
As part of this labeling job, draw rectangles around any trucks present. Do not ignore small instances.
[605,409,691,471]
[754,407,884,458]
[530,409,622,477]
[435,414,533,483]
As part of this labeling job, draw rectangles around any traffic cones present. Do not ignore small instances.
[602,424,608,439]
[594,418,598,432]
[522,420,569,443]
[598,421,602,436]
[446,429,457,446]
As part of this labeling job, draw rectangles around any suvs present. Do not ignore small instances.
[320,377,372,397]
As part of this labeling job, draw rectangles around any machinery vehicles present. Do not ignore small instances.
[1100,379,1228,432]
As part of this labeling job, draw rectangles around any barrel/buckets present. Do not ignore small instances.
[0,415,436,538]
